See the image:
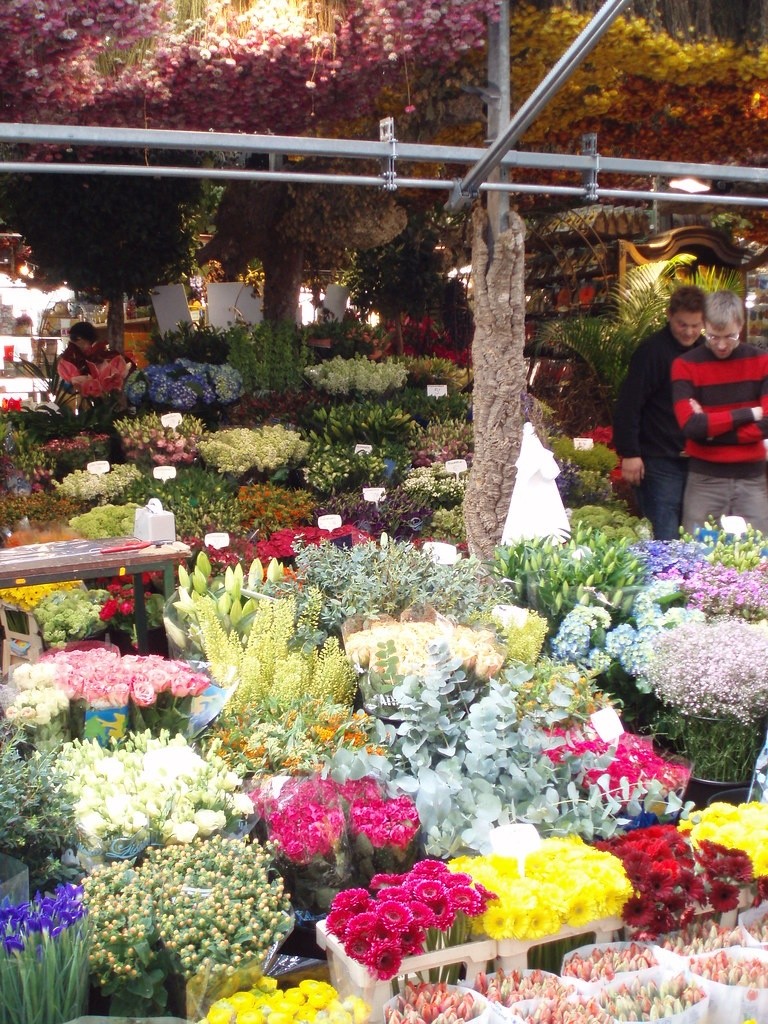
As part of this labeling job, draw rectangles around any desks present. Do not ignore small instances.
[0,535,193,655]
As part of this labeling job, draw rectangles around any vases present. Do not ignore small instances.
[108,624,165,657]
[707,789,763,805]
[676,714,768,810]
[362,703,408,737]
[185,407,219,433]
[294,907,327,961]
[65,629,105,641]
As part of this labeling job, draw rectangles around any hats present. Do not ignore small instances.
[70,322,105,342]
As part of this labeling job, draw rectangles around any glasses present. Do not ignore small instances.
[91,341,97,348]
[706,334,740,345]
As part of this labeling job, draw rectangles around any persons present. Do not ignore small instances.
[59,321,99,374]
[613,287,706,540]
[671,291,768,540]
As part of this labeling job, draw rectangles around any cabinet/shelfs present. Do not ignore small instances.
[0,233,124,404]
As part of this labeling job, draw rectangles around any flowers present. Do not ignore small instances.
[0,307,651,540]
[0,516,768,1024]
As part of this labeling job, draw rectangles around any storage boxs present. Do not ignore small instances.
[315,919,497,1024]
[493,912,625,972]
[0,599,43,646]
[625,888,749,947]
[2,639,45,676]
